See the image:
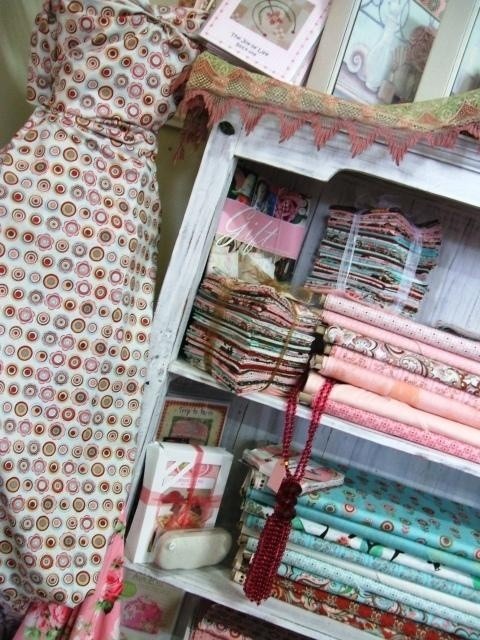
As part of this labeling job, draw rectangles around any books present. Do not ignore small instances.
[243,445,345,494]
[157,396,231,448]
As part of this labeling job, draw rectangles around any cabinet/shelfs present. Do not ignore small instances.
[106,89,479,639]
